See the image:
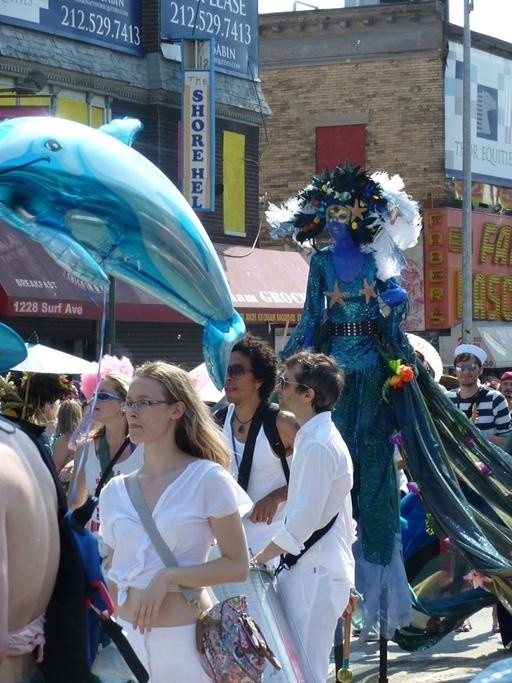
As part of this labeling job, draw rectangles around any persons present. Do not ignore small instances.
[266,170,420,683]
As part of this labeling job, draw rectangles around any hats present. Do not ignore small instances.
[500,371,512,381]
[454,343,488,367]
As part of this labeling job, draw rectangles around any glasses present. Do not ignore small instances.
[227,364,255,380]
[119,397,176,412]
[91,391,124,402]
[277,374,311,393]
[455,363,479,373]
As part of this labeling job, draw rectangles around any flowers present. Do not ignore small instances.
[380,359,415,404]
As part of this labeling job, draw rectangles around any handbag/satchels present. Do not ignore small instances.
[71,493,97,526]
[192,592,283,682]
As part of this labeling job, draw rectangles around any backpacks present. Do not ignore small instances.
[0,409,116,683]
